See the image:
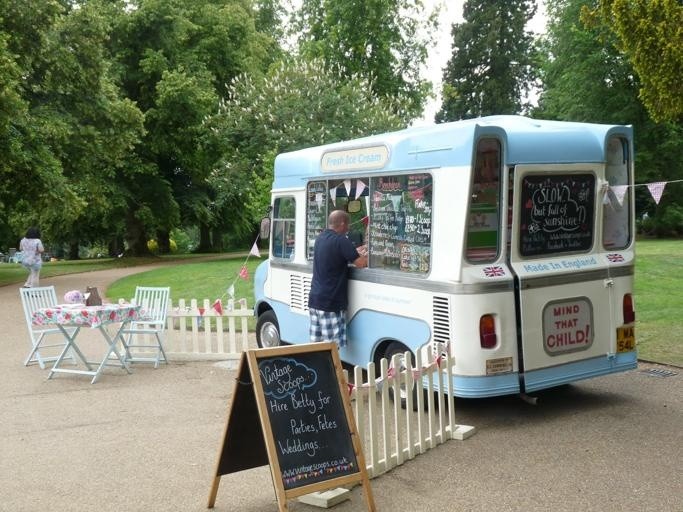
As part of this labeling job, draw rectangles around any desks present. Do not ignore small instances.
[32,300,145,385]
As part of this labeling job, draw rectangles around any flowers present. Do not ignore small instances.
[64,290,82,304]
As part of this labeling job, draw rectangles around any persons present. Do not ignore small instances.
[19,227,45,298]
[308,210,368,350]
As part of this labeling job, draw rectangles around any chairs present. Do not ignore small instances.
[15,285,76,371]
[114,283,172,370]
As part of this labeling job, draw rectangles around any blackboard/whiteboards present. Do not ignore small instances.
[369,173,433,274]
[520,173,595,256]
[245,341,366,500]
[304,180,327,261]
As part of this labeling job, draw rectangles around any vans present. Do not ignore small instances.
[249,113,639,405]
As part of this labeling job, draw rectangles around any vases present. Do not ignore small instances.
[72,299,85,308]
